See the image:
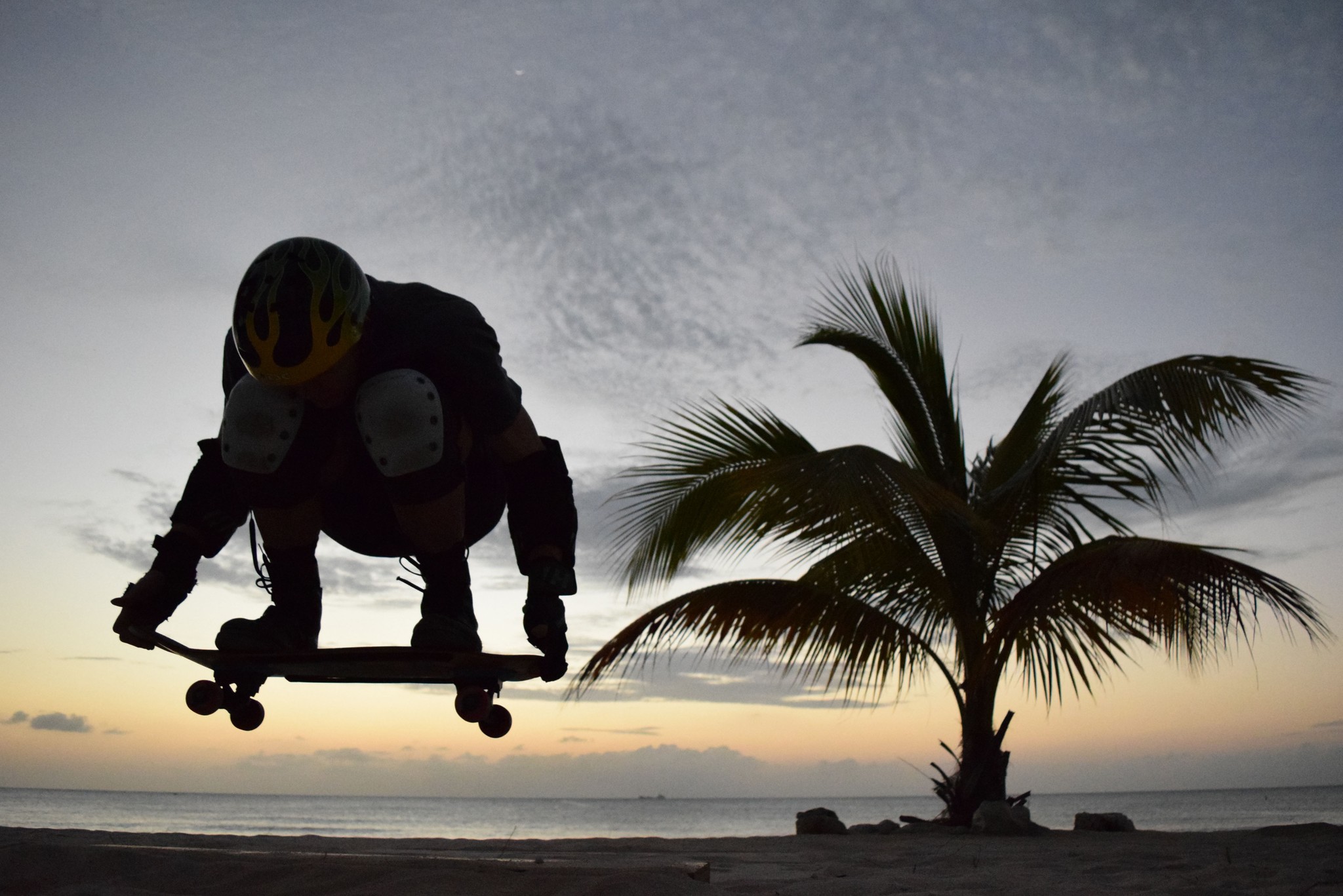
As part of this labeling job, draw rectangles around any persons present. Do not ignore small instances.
[113,237,579,655]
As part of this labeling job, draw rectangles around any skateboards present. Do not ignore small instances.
[154,632,545,739]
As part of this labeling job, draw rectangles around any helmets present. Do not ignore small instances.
[231,234,371,383]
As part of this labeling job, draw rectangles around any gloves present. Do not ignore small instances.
[522,595,569,664]
[109,535,199,651]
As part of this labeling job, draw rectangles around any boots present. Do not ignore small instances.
[397,545,482,651]
[213,554,323,654]
